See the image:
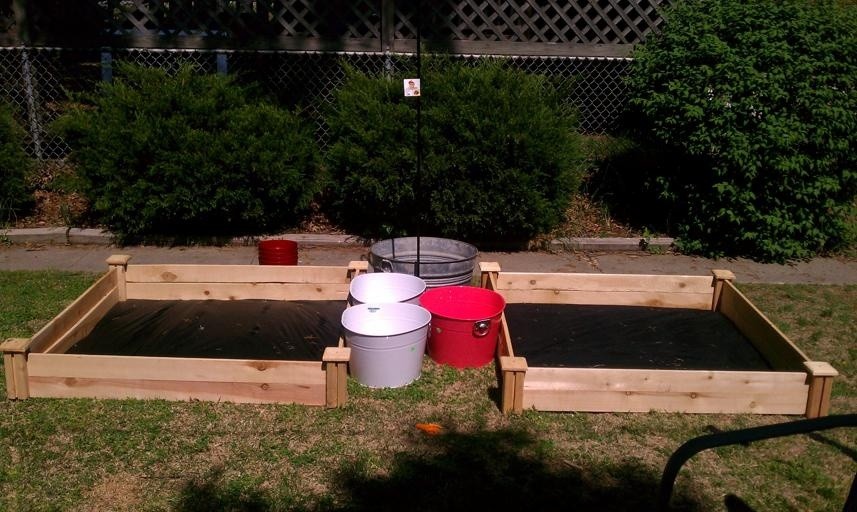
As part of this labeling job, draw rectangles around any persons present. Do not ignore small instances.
[407,81,418,95]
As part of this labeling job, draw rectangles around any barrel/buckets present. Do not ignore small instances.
[418,285,507,367]
[368,236,479,288]
[340,302,432,390]
[258,239,299,264]
[350,272,425,305]
